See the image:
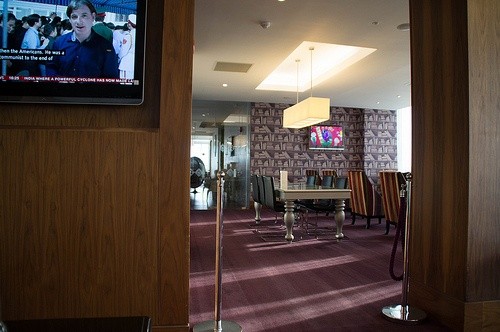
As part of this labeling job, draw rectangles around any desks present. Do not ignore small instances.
[274,182,352,244]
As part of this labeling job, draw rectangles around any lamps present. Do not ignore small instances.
[283,45,330,129]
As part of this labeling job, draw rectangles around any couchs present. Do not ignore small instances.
[347,170,385,230]
[306,169,352,218]
[379,171,408,235]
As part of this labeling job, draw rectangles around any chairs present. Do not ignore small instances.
[250,174,309,240]
[291,175,348,239]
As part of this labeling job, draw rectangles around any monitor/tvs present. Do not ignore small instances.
[308,125,344,151]
[0,0,147,106]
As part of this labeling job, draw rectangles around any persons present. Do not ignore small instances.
[0,2,145,80]
